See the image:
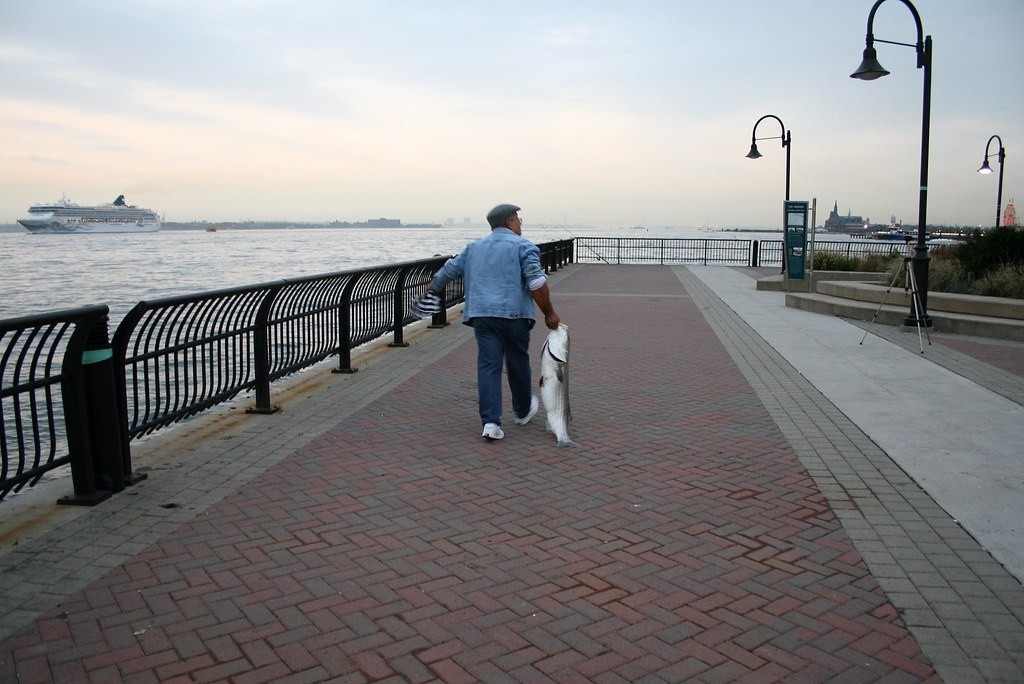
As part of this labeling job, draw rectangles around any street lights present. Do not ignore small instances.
[849,0,932,327]
[976,134,1006,228]
[744,115,791,274]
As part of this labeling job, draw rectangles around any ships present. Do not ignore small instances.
[16,192,162,233]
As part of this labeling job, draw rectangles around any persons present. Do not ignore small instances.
[430,204,561,439]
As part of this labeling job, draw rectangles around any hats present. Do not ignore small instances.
[487,204,521,228]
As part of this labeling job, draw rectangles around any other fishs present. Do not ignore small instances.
[538,323,583,449]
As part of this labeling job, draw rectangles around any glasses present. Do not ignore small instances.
[510,218,523,223]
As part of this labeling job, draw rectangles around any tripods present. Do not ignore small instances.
[859,236,932,355]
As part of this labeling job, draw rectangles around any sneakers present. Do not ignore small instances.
[515,394,538,425]
[482,422,504,439]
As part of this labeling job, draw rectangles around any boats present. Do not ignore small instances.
[877,220,931,241]
[807,228,828,233]
